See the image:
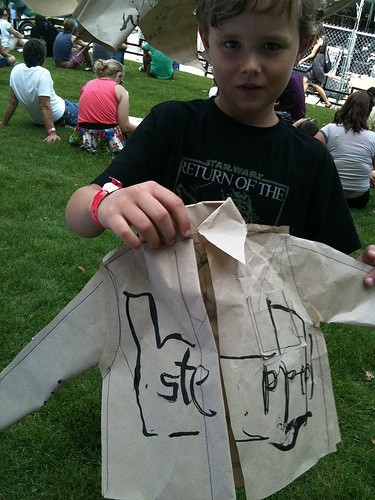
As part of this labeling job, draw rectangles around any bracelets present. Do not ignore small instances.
[90,177,122,227]
[47,128,55,133]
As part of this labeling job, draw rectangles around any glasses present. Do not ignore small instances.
[3,13,9,16]
[118,78,123,85]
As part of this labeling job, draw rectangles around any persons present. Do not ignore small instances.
[0,0,179,155]
[274,32,375,208]
[64,0,375,287]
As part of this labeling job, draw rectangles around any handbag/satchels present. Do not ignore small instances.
[324,51,331,74]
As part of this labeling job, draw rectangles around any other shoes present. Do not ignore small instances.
[325,103,332,108]
[83,66,93,71]
[139,65,145,72]
